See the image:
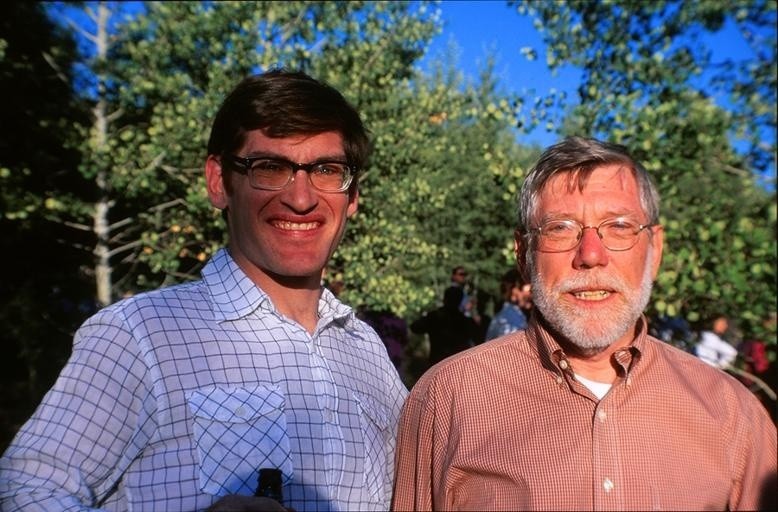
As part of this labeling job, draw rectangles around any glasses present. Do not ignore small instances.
[219,149,362,193]
[526,214,657,252]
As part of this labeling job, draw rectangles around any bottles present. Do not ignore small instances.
[253,467,287,508]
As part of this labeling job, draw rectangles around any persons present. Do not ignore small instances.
[388,137,778,511]
[443,264,482,358]
[733,318,771,399]
[0,67,413,511]
[685,313,739,374]
[483,268,536,346]
[408,284,476,373]
